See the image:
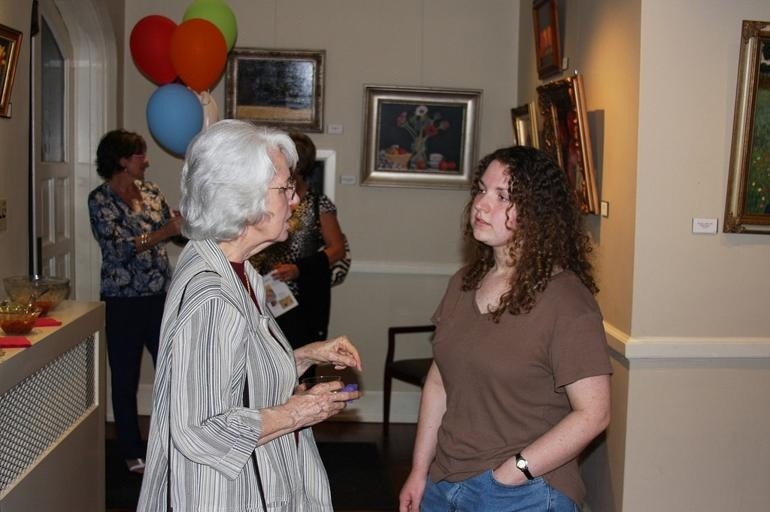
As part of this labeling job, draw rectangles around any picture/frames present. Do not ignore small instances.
[532,0,562,79]
[723,20,770,234]
[536,73,600,216]
[223,47,326,133]
[511,102,539,150]
[315,149,337,204]
[358,84,484,191]
[0,22,23,118]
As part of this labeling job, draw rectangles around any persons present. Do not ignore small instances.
[88,128,185,476]
[138,118,363,512]
[252,132,344,386]
[398,145,612,512]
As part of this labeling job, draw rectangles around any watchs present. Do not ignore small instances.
[515,453,534,481]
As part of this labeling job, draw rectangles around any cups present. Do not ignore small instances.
[302,375,341,395]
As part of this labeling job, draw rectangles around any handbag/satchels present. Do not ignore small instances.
[305,230,353,288]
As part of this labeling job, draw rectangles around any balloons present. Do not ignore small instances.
[146,82,203,158]
[183,0,237,54]
[168,19,227,92]
[129,15,179,84]
[186,87,218,128]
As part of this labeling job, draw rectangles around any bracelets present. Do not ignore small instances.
[141,233,148,251]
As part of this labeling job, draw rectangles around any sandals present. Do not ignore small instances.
[116,454,146,475]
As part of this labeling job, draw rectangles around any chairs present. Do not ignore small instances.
[383,325,436,433]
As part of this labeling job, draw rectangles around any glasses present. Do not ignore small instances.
[266,180,299,199]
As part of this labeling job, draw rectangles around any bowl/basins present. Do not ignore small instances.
[3,274,70,316]
[0,304,43,335]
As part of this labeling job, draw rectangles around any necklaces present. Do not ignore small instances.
[243,270,252,296]
